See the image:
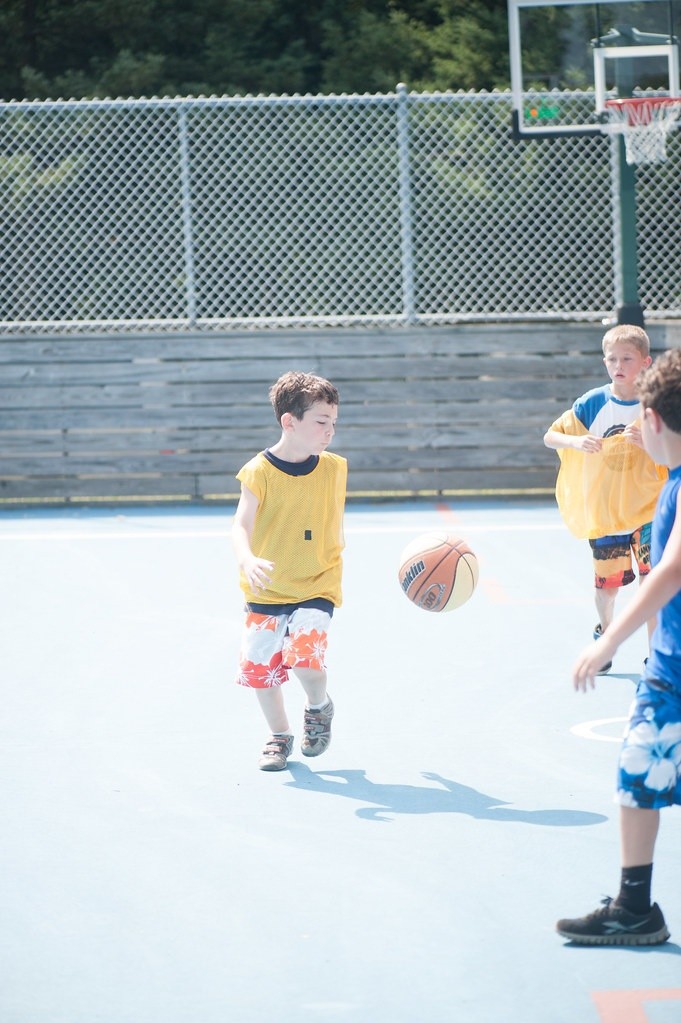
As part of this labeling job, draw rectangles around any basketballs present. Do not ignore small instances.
[399,533,479,612]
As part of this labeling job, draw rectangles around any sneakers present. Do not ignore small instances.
[301,692,334,757]
[259,734,294,771]
[557,896,670,945]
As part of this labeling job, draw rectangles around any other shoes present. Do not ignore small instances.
[594,623,613,675]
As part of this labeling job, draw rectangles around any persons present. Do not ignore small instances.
[555,350,681,948]
[233,371,348,772]
[543,324,670,676]
